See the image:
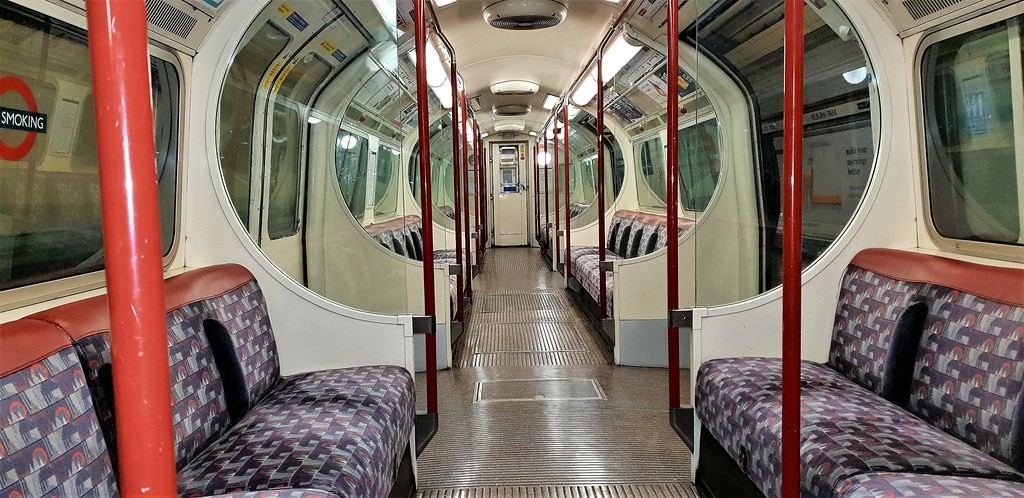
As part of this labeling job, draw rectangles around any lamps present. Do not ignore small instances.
[373,0,644,151]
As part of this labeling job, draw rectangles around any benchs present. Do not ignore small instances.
[694,245,1024,491]
[363,214,473,323]
[439,206,456,220]
[537,201,591,250]
[565,210,699,319]
[1,263,417,496]
